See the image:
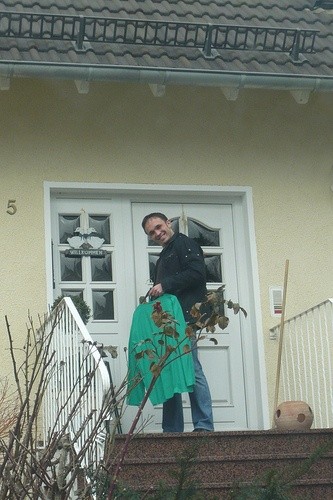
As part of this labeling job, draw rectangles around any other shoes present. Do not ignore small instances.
[192,427,213,435]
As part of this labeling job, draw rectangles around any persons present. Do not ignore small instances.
[141,212,215,432]
